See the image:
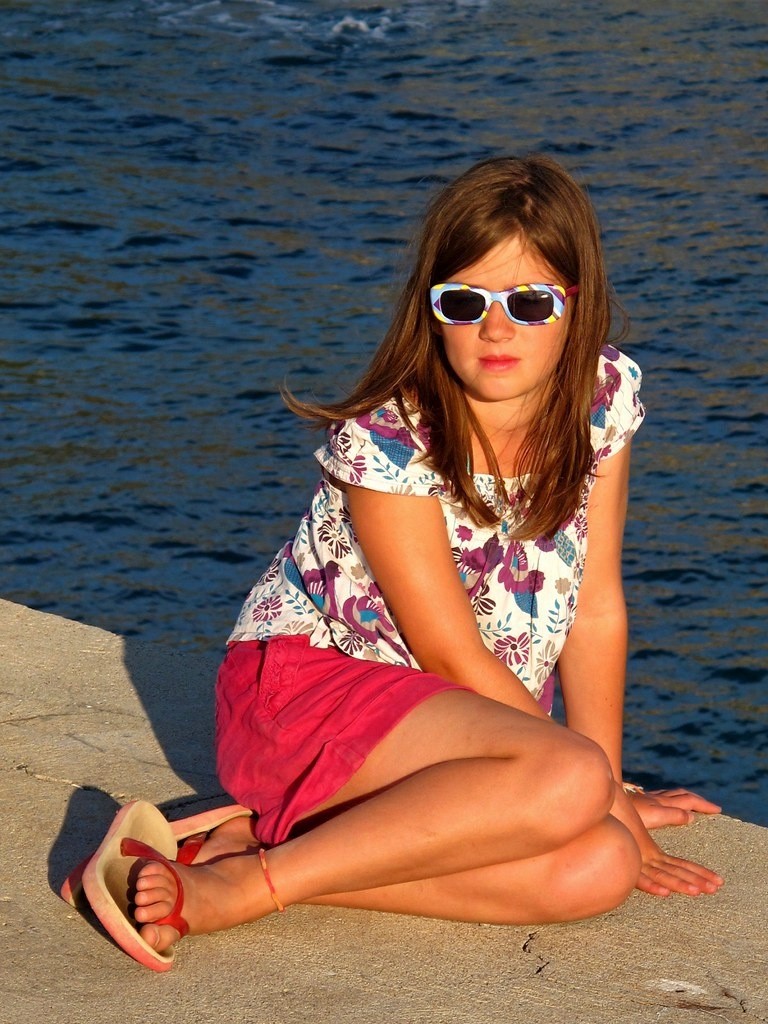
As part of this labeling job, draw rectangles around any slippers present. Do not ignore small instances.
[61,803,253,909]
[82,800,190,972]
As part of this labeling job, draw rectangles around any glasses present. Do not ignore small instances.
[430,283,580,325]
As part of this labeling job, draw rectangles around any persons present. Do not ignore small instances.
[59,157,724,974]
